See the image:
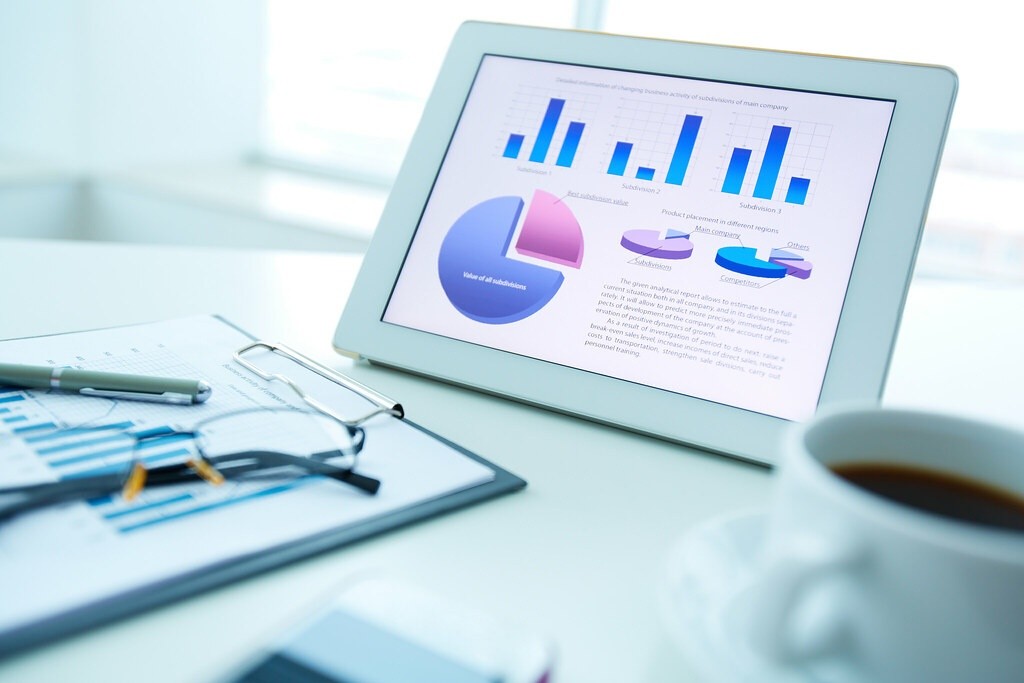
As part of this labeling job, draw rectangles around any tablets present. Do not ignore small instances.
[333,20,959,469]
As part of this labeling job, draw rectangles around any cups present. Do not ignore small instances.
[752,405,1024,683]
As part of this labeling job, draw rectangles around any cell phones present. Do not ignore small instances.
[214,575,550,683]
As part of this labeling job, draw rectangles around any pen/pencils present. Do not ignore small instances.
[0,363,211,406]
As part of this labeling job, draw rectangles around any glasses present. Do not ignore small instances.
[0,406,381,524]
